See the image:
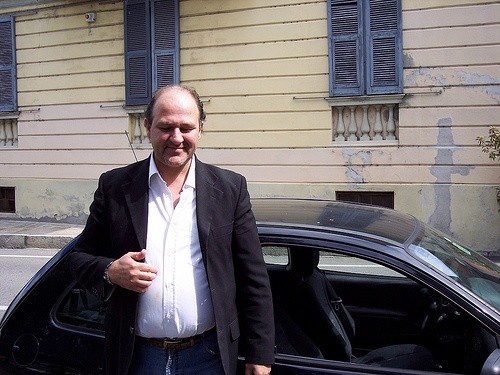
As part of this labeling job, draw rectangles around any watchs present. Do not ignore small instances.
[102,260,114,286]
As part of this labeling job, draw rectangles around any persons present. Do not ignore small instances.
[67,82,276,375]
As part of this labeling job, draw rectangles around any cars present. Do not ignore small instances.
[0,200,500,375]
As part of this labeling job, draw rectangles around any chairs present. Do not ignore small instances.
[285,247,435,371]
[272,297,323,359]
